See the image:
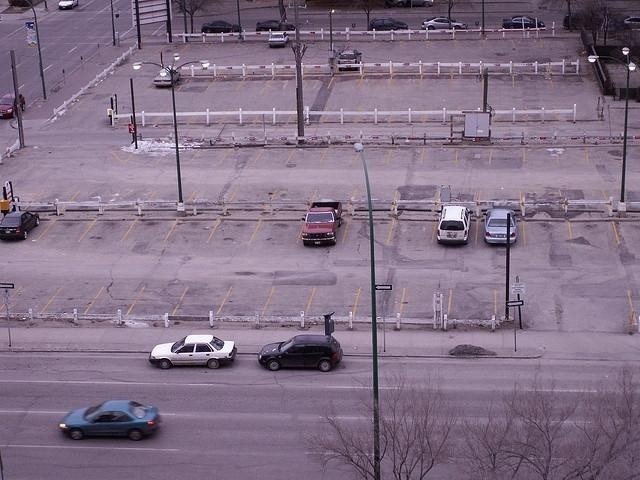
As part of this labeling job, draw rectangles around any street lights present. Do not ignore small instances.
[132,53,210,217]
[353,143,392,480]
[588,47,637,218]
[328,8,335,65]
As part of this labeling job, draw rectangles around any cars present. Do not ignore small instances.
[337,50,362,71]
[201,21,239,33]
[58,400,159,441]
[502,16,544,29]
[0,93,25,119]
[149,335,343,372]
[152,72,180,86]
[0,211,39,240]
[369,0,467,31]
[437,206,519,244]
[59,0,78,9]
[624,17,640,28]
[256,20,295,48]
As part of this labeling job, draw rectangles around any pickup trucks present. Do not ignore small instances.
[301,201,342,246]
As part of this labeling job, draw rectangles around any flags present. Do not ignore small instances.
[25,20,39,49]
